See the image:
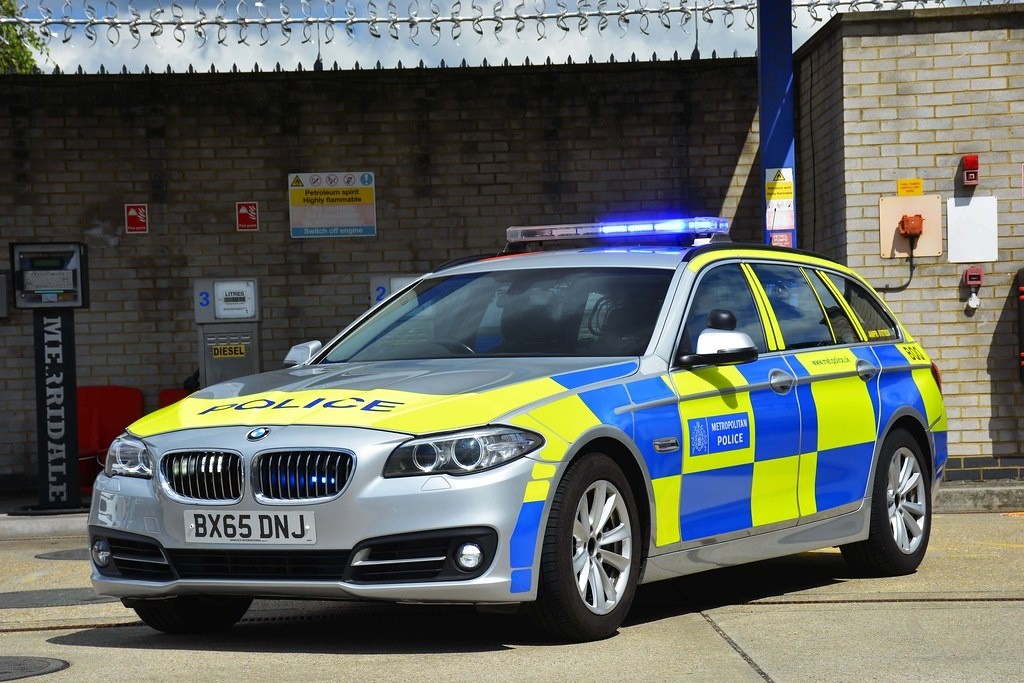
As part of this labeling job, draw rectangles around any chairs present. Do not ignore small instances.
[480,272,583,358]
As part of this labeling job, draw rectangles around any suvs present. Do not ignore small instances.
[89,214,950,639]
